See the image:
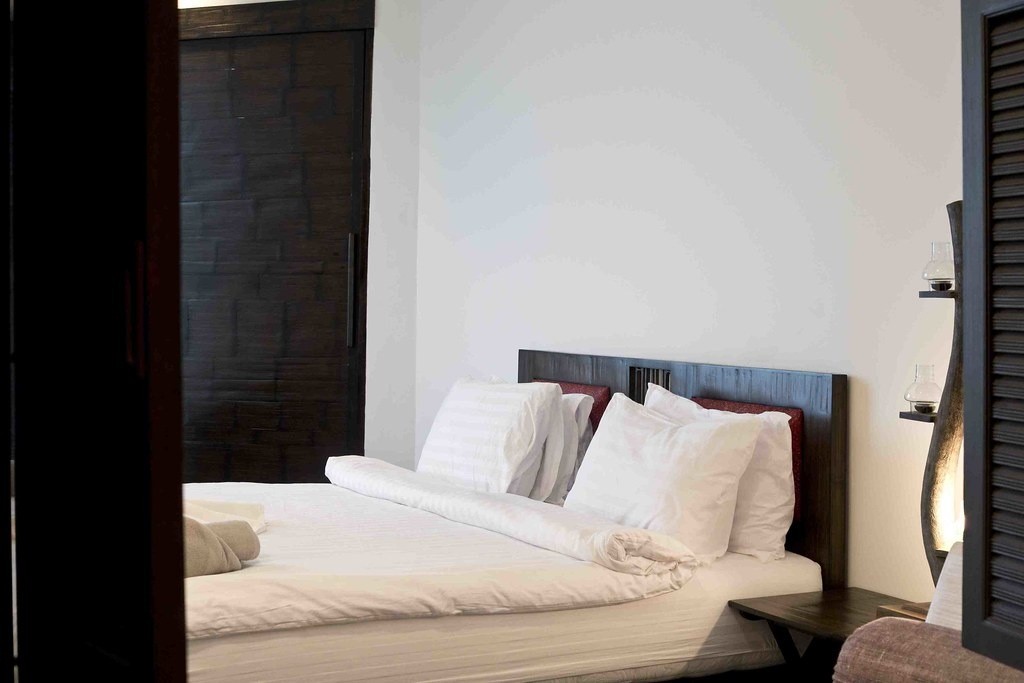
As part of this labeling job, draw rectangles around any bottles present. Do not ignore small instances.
[920,240,956,291]
[904,364,942,415]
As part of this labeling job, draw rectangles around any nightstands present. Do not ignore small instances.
[728,586,916,683]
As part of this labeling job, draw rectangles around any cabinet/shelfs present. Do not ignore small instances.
[900,290,955,423]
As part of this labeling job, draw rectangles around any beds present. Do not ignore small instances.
[171,349,849,683]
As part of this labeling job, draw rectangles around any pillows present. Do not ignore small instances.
[416,374,565,501]
[563,392,764,566]
[643,382,795,564]
[488,375,594,504]
